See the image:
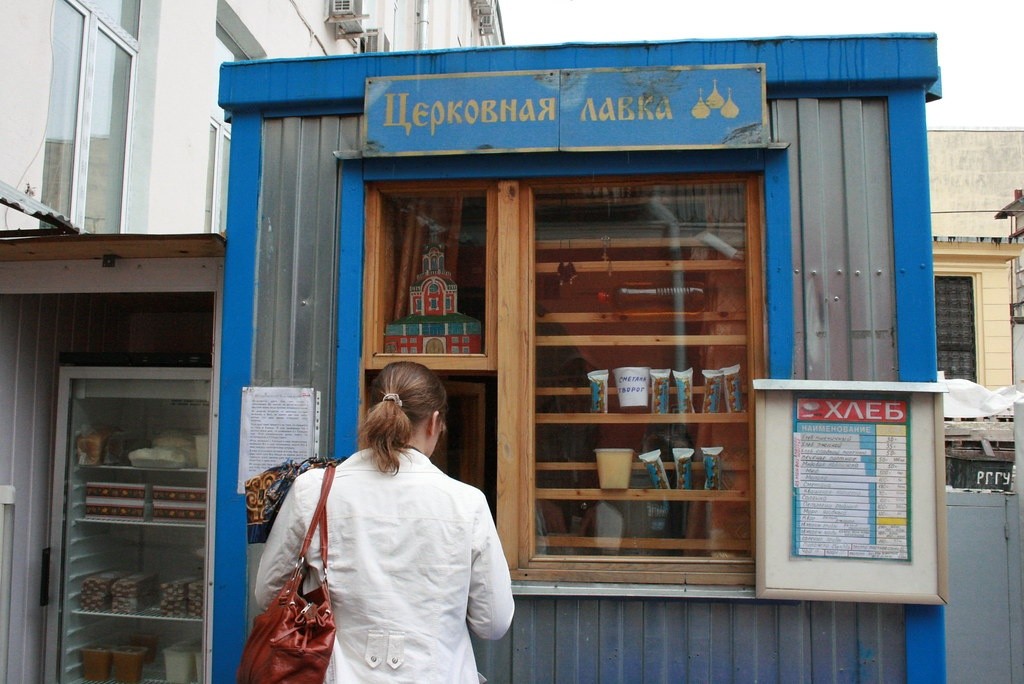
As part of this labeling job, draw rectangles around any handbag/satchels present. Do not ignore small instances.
[236,461,336,684]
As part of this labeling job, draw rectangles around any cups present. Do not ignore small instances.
[164,640,203,684]
[81,632,158,682]
[193,435,210,469]
[594,448,634,490]
[612,366,650,411]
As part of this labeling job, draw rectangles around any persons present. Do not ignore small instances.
[535,301,602,554]
[255,360,514,684]
